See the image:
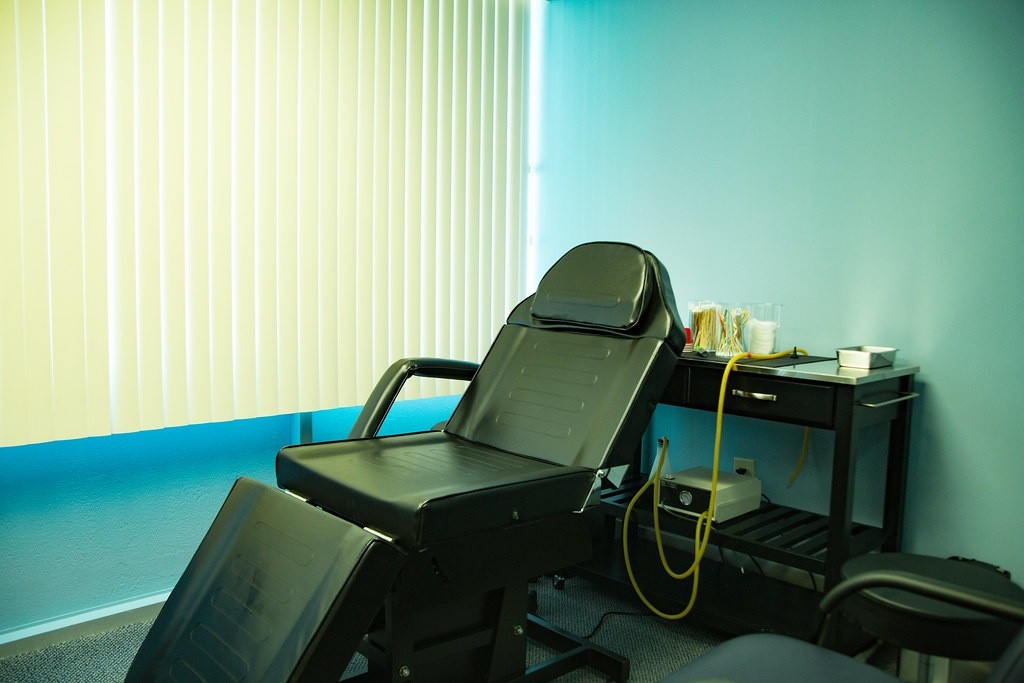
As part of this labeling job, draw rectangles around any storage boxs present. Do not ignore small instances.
[836,345,900,369]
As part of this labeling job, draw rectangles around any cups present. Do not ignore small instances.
[715,303,749,358]
[751,303,780,355]
[688,300,718,352]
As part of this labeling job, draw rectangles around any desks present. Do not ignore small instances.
[590,355,922,656]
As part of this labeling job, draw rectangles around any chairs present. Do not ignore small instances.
[123,239,687,683]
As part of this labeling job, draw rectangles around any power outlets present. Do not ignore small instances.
[733,456,755,478]
